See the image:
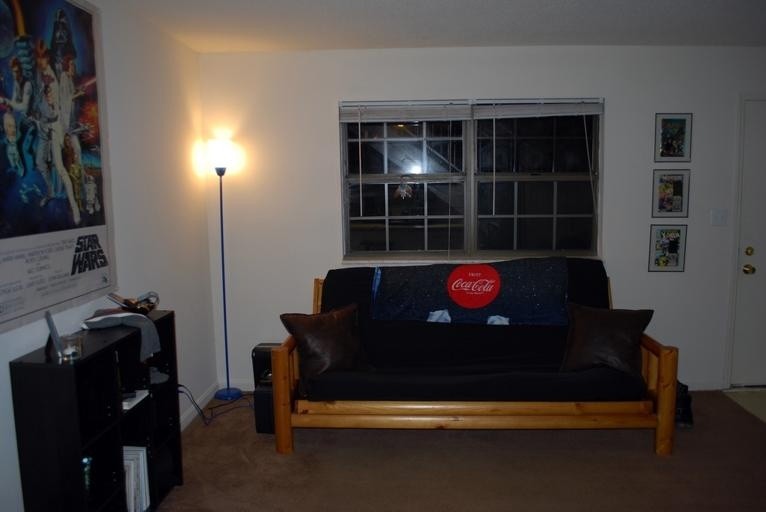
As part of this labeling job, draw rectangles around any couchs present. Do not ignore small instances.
[272,256,679,455]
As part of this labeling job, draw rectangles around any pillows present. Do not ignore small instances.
[556,301,654,381]
[278,300,362,384]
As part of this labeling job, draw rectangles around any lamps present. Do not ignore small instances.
[206,140,243,401]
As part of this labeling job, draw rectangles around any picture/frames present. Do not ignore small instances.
[0,0,119,334]
[647,113,693,272]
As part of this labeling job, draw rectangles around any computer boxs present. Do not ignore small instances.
[252,342,284,386]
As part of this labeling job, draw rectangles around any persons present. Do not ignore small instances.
[662,122,679,157]
[667,232,680,266]
[0,38,95,225]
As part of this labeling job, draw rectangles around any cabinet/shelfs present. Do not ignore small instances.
[10,310,184,510]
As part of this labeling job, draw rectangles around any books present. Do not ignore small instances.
[123,445,150,511]
[122,388,150,410]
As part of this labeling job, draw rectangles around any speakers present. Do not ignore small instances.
[253,385,275,434]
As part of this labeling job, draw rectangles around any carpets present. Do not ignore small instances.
[157,390,766,512]
